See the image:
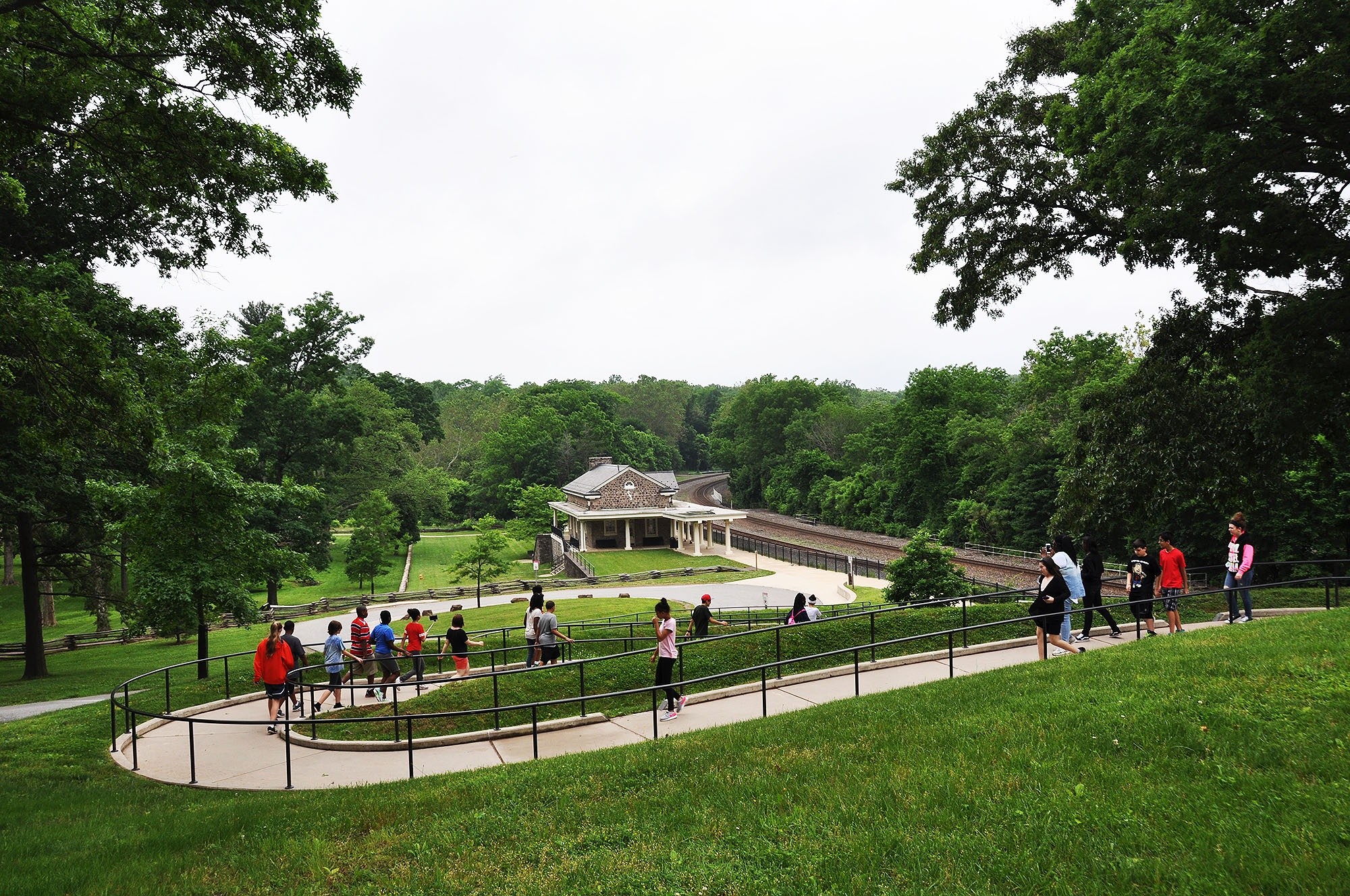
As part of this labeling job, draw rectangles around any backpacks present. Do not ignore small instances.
[788,608,805,624]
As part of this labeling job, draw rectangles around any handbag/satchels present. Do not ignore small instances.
[1028,593,1053,627]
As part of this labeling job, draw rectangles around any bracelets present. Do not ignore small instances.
[686,630,691,632]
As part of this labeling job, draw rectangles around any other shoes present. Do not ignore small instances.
[373,688,391,702]
[314,702,322,712]
[365,689,375,697]
[267,726,278,733]
[277,709,285,717]
[1078,647,1086,653]
[415,684,429,689]
[292,700,304,711]
[395,677,401,691]
[1144,630,1157,638]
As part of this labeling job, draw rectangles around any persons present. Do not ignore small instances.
[1027,555,1086,661]
[341,605,378,698]
[276,620,309,717]
[437,613,484,679]
[395,608,428,691]
[1074,536,1122,642]
[369,610,413,702]
[534,600,575,666]
[523,585,544,668]
[685,594,728,640]
[1157,530,1189,633]
[804,594,822,621]
[313,620,363,712]
[784,593,811,625]
[1125,538,1162,636]
[1039,534,1086,656]
[1223,512,1255,624]
[253,620,295,734]
[649,597,689,722]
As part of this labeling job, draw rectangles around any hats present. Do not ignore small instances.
[701,594,715,601]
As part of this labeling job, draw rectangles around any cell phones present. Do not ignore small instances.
[1046,544,1051,553]
[430,615,437,620]
[1042,596,1049,600]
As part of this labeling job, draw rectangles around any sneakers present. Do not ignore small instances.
[1052,647,1070,655]
[1068,636,1076,644]
[1109,627,1122,637]
[1075,633,1090,642]
[659,710,677,721]
[1223,615,1254,624]
[676,695,688,713]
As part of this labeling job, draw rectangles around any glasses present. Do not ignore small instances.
[1159,539,1165,542]
